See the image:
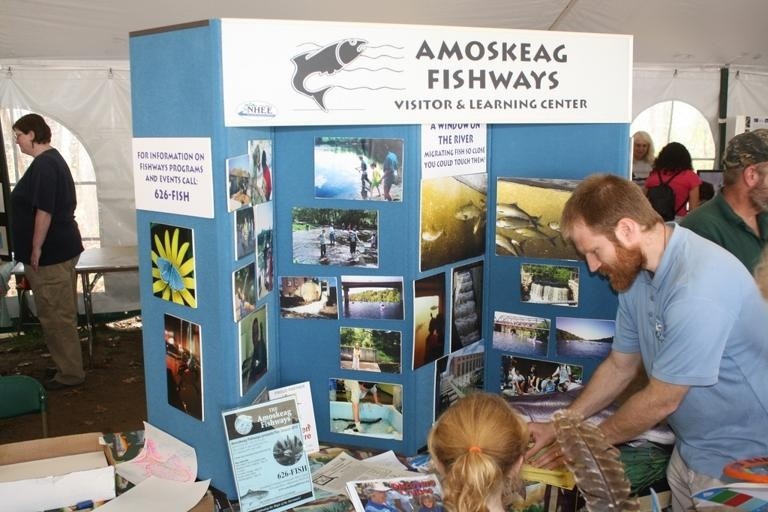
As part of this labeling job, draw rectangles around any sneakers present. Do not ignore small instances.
[46,379,86,391]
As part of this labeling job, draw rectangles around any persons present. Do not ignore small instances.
[418,493,445,511]
[3,112,87,390]
[237,141,400,433]
[365,480,403,511]
[631,129,655,184]
[506,360,583,397]
[427,392,522,511]
[382,483,414,511]
[515,174,768,511]
[641,141,702,219]
[680,127,768,276]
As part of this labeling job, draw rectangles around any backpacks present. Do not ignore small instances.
[647,169,691,221]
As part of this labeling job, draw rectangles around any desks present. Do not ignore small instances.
[0,244,138,370]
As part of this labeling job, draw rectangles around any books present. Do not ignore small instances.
[519,439,580,491]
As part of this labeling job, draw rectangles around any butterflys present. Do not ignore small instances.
[157,257,185,292]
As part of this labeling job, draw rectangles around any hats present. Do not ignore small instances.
[719,128,768,171]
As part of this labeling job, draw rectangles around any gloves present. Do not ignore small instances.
[355,422,363,433]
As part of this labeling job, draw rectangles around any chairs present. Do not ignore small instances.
[0,374,48,445]
